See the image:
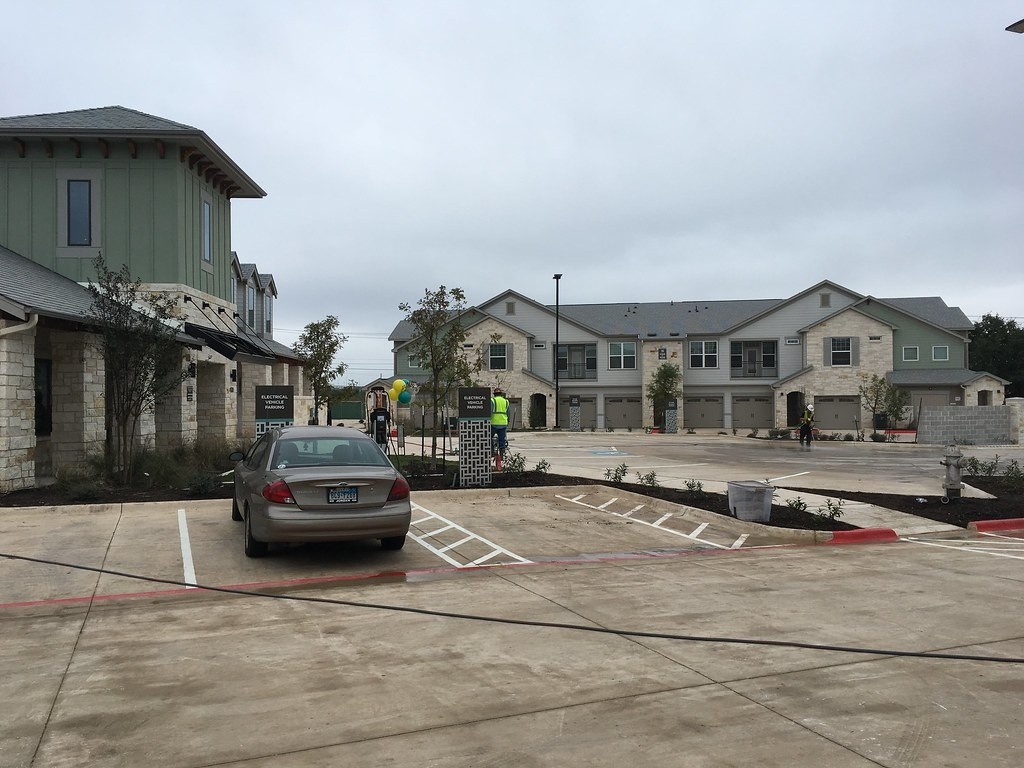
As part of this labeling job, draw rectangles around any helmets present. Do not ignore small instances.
[807,404,814,411]
[493,388,502,394]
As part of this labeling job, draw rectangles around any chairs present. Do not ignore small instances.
[332,444,353,462]
[279,443,299,464]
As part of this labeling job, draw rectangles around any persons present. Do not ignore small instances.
[799,404,815,446]
[490,388,511,456]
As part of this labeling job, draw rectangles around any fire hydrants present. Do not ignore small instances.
[940,445,971,497]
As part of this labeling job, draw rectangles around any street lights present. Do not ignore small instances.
[551,273,562,431]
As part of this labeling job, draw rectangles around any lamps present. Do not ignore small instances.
[230,369,236,382]
[188,363,196,378]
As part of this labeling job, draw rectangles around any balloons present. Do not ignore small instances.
[388,378,411,404]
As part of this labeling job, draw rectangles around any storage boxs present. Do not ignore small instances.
[727,480,775,523]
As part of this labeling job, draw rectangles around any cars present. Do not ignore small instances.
[229,425,412,559]
